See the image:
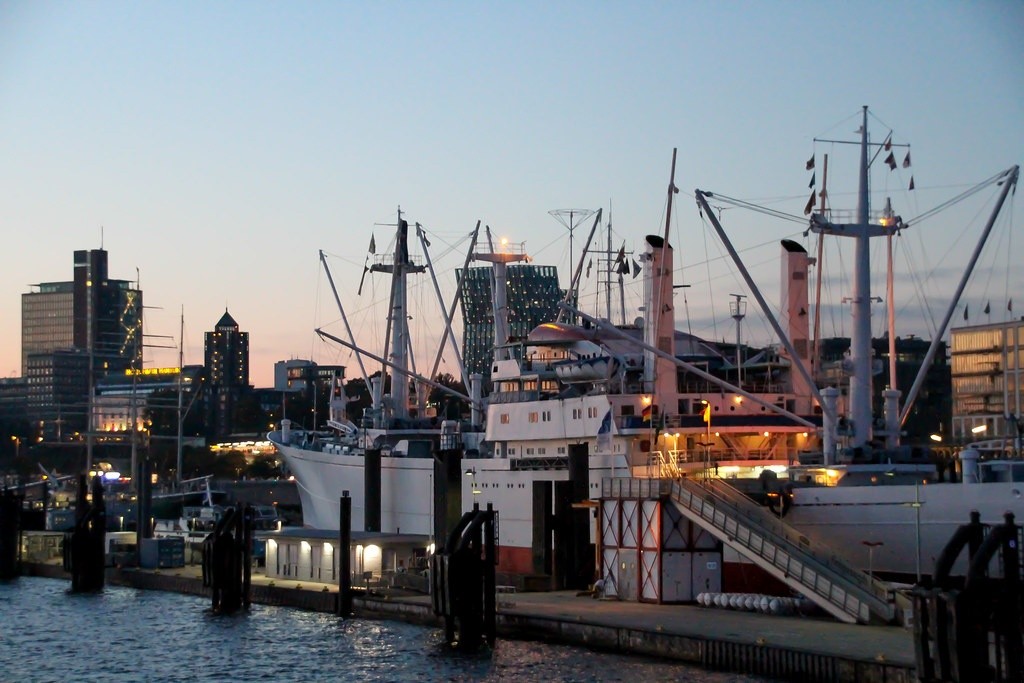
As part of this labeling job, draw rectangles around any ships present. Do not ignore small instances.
[267,104,1024,596]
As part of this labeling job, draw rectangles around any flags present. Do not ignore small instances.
[641,405,652,422]
[884,137,915,191]
[615,246,642,279]
[701,402,710,422]
[964,299,1012,320]
[803,153,817,215]
[596,409,619,450]
[368,234,376,253]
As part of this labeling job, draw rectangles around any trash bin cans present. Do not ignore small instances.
[253,541,265,556]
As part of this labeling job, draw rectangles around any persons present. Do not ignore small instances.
[934,449,959,482]
